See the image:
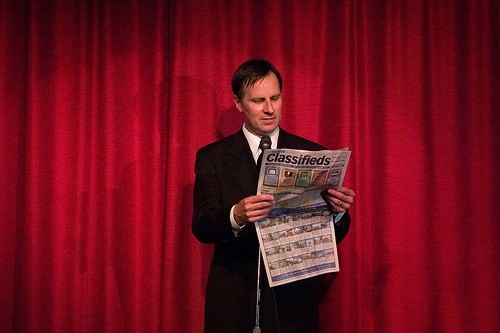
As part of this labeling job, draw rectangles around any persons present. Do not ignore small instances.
[192,58,355,333]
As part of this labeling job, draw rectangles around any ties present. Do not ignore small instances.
[257,141,264,171]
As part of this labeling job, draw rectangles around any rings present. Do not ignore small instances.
[339,201,343,206]
